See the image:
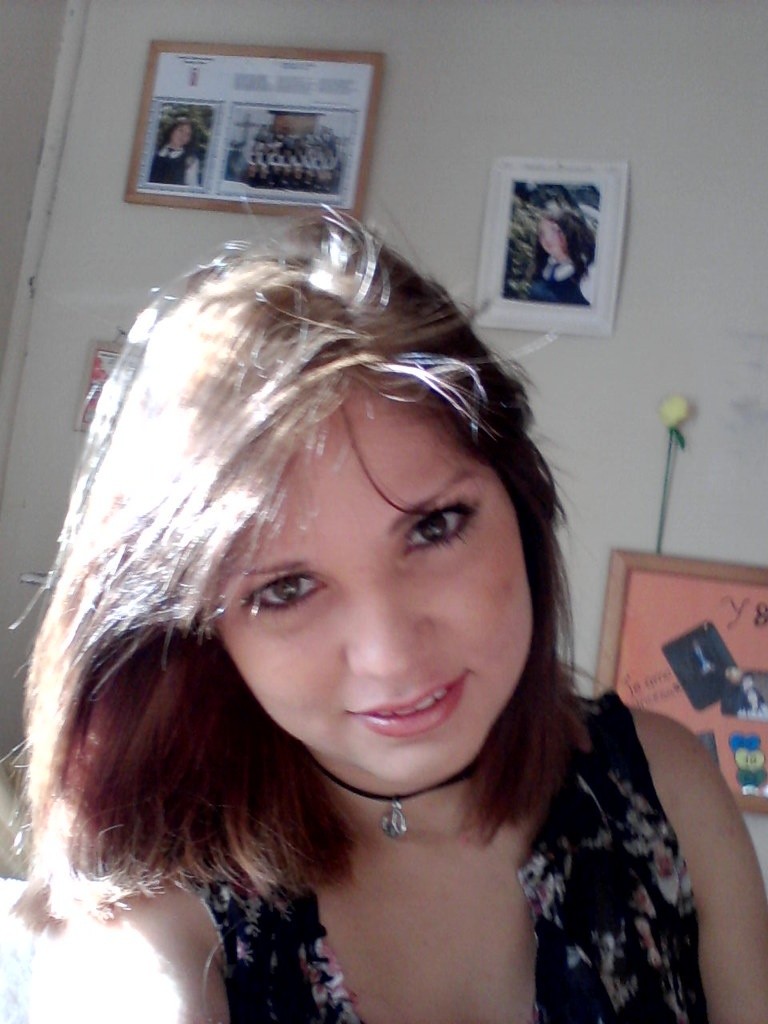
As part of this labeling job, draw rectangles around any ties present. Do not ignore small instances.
[548,264,558,279]
[165,148,175,158]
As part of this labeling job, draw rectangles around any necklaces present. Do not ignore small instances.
[313,752,474,836]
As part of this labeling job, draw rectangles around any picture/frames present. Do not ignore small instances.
[123,39,389,223]
[595,546,768,810]
[474,154,632,339]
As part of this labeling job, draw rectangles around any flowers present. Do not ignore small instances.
[655,396,689,553]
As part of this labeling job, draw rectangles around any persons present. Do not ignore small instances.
[147,118,200,186]
[244,123,345,194]
[528,208,597,308]
[721,666,768,721]
[0,238,768,1024]
